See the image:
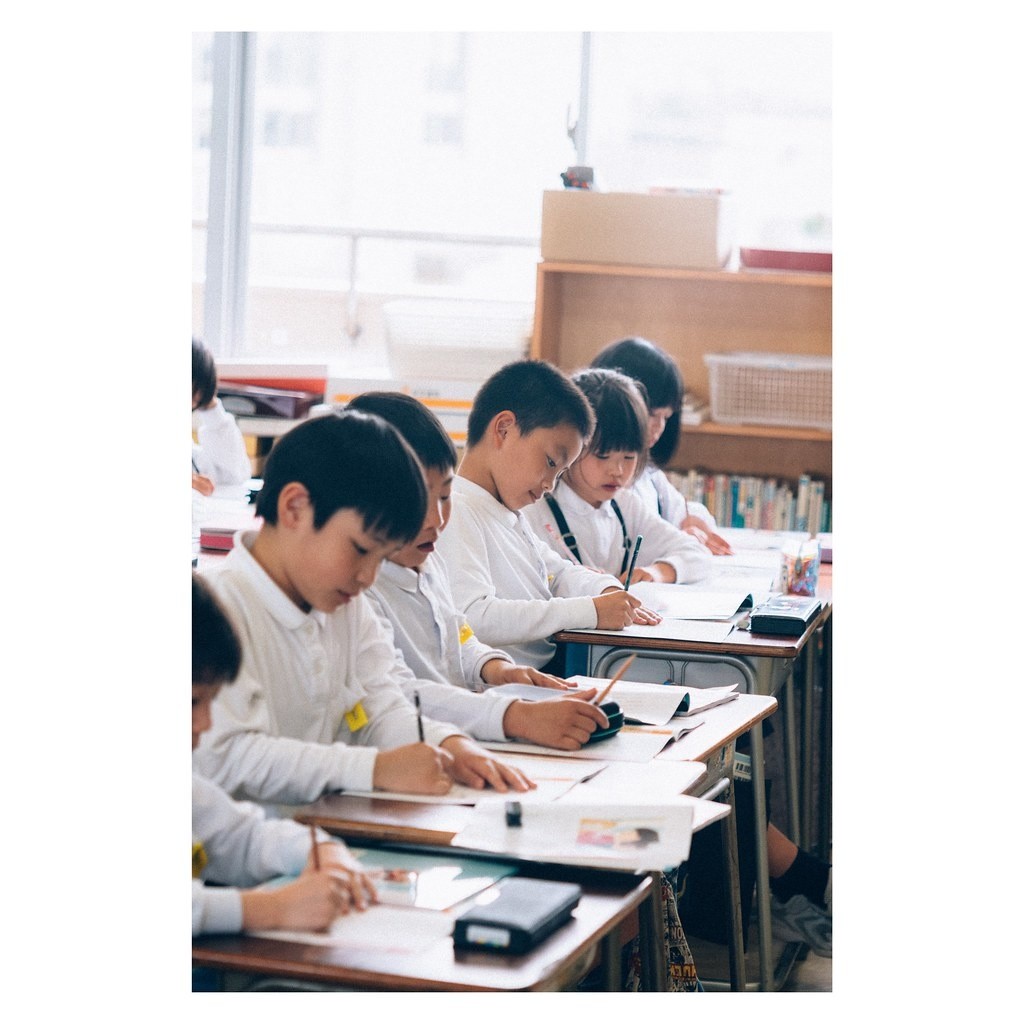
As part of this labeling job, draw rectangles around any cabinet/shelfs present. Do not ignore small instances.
[530,261,834,500]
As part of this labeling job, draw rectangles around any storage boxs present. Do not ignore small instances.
[701,353,832,433]
[541,190,732,271]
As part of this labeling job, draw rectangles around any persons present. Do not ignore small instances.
[193,567,387,994]
[326,390,611,749]
[588,334,733,555]
[204,409,540,819]
[520,366,715,586]
[430,358,831,959]
[192,335,251,505]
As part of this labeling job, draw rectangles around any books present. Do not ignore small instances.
[561,549,784,643]
[678,391,713,426]
[339,675,740,877]
[658,470,831,535]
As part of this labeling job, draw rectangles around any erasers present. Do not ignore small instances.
[736,620,749,629]
[505,801,522,827]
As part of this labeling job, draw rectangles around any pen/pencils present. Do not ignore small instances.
[311,821,320,869]
[414,689,425,742]
[624,535,643,591]
[545,523,581,565]
[685,496,689,516]
[192,460,199,474]
[593,653,637,706]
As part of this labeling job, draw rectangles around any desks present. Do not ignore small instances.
[773,561,833,852]
[267,750,710,950]
[555,600,828,992]
[192,846,653,992]
[474,674,778,992]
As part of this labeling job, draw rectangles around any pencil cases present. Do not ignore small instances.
[483,682,624,748]
[200,528,237,551]
[749,595,822,637]
[453,877,582,964]
